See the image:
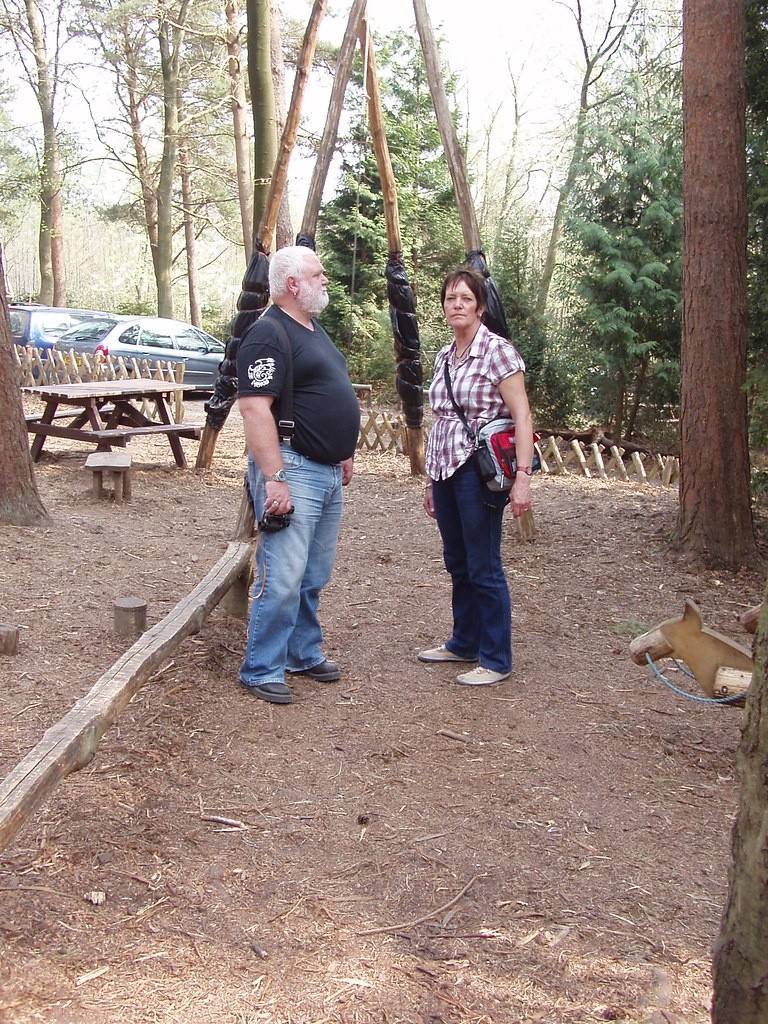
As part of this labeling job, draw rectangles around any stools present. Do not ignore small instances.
[84,452,133,504]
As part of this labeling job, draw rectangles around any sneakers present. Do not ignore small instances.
[417,644,479,662]
[457,667,510,685]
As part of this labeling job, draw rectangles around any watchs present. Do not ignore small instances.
[263,469,286,481]
[516,466,534,477]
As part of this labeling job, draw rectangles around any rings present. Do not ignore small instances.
[274,501,280,509]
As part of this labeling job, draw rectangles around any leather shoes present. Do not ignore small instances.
[285,660,340,681]
[240,680,292,703]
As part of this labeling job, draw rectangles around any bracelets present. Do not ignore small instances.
[423,481,432,490]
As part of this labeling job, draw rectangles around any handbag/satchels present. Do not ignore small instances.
[473,416,541,491]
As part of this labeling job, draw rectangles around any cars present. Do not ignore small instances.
[43,315,225,391]
[8,299,119,377]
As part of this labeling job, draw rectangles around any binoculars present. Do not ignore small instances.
[254,504,296,532]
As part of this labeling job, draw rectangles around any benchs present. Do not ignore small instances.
[25,405,115,422]
[82,423,205,439]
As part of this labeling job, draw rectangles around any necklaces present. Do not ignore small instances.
[454,323,481,359]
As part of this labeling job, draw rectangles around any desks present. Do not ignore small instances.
[20,378,196,469]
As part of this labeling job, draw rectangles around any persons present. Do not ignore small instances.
[417,268,534,687]
[235,245,361,703]
